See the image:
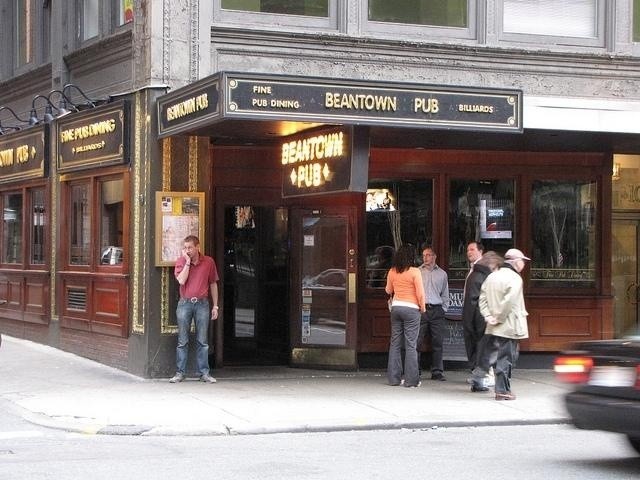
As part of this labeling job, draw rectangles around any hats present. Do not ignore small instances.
[504,248,533,261]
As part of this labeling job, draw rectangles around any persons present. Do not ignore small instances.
[169,235,219,383]
[384,243,426,388]
[368,245,395,287]
[380,192,391,209]
[461,249,505,393]
[469,246,532,401]
[461,240,496,387]
[415,246,450,382]
[456,185,477,216]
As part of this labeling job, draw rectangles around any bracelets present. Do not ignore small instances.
[185,263,190,266]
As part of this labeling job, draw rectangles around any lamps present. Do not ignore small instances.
[0,83,99,134]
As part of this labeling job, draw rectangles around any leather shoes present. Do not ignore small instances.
[472,383,490,392]
[432,373,447,381]
[496,392,517,401]
[200,374,217,383]
[169,374,186,383]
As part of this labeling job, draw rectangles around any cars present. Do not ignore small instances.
[377,247,394,258]
[366,254,386,286]
[554,323,640,448]
[102,246,122,264]
[304,269,346,325]
[447,261,470,279]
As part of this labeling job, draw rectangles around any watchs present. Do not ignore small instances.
[213,305,218,309]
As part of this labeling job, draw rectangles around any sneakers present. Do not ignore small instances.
[411,381,422,388]
[400,379,406,386]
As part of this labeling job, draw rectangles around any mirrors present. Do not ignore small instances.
[301,216,348,345]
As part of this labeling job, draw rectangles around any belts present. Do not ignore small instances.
[426,304,442,308]
[187,297,209,303]
